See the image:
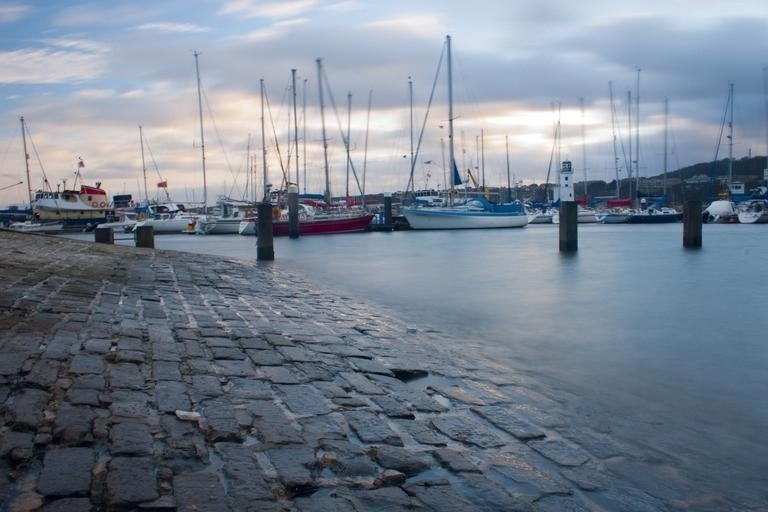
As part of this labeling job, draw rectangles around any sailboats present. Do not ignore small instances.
[0,47,378,237]
[368,31,768,231]
